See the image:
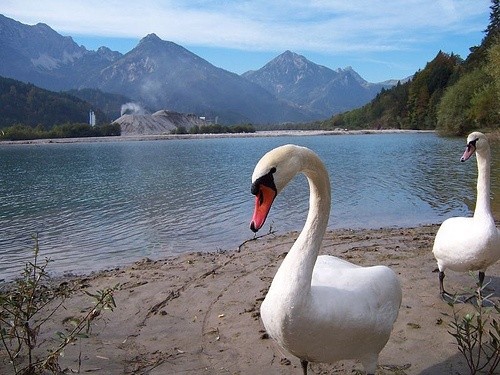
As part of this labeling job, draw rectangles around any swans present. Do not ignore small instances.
[432,130,500,314]
[250,144,402,375]
